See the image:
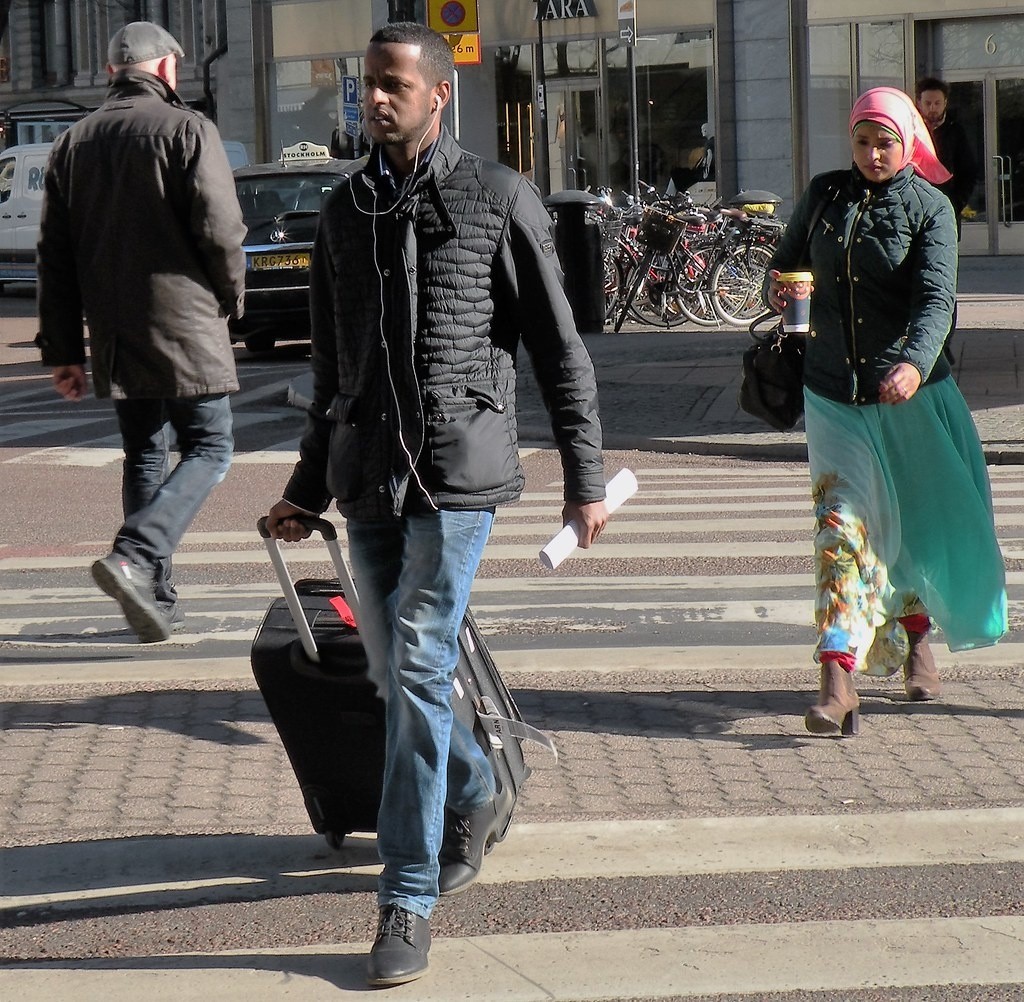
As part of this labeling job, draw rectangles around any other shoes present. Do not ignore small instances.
[91,552,185,644]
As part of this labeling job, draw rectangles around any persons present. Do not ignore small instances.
[265,21,611,989]
[760,85,1008,737]
[912,77,981,366]
[33,19,249,645]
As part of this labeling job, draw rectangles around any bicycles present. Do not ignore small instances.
[544,179,790,333]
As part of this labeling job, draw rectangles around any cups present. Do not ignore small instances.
[775,272,814,333]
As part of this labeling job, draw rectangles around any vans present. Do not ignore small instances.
[0,138,249,283]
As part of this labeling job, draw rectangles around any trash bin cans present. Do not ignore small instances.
[544,189,609,334]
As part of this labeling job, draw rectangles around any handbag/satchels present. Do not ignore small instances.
[737,310,805,433]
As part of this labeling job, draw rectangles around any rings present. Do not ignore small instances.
[903,388,907,393]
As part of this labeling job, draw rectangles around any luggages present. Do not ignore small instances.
[251,513,532,856]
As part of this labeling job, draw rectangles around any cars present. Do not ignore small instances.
[228,141,371,354]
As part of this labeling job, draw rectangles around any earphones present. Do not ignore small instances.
[435,95,442,111]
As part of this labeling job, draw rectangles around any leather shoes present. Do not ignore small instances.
[437,774,502,896]
[364,902,431,986]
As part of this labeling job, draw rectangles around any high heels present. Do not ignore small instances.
[803,660,859,735]
[903,630,942,700]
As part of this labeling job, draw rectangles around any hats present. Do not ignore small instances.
[107,22,185,65]
